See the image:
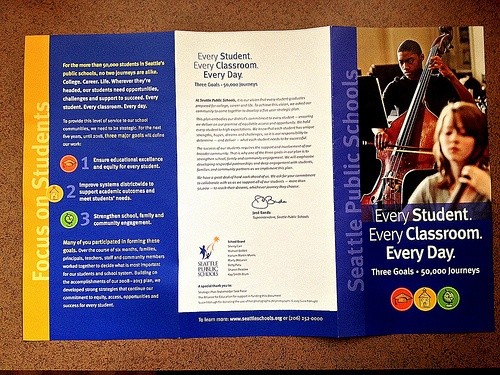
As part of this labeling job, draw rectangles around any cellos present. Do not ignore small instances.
[357,25,462,221]
[437,154,491,222]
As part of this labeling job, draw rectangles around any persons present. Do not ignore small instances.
[369,38,475,151]
[396,101,493,218]
